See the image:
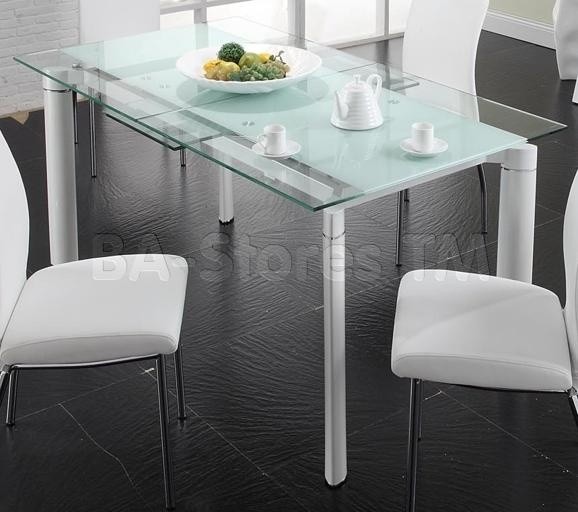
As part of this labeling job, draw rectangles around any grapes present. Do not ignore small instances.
[229,50,289,81]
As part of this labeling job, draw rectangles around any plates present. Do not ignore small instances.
[176,42,322,94]
[400,137,449,158]
[251,139,302,159]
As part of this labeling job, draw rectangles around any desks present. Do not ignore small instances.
[14,17,568,489]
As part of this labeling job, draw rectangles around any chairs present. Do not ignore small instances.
[72,0,187,176]
[328,0,489,266]
[0,128,189,510]
[391,168,578,512]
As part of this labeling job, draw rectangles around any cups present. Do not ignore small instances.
[256,124,287,155]
[411,121,434,153]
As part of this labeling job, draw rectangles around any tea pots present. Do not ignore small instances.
[329,74,384,131]
